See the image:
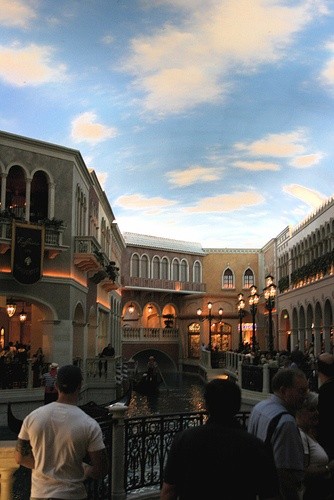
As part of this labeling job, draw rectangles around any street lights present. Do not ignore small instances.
[234,293,246,353]
[246,284,261,352]
[262,274,277,353]
[197,302,224,350]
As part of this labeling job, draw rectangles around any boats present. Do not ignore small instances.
[7,401,113,435]
[131,368,157,393]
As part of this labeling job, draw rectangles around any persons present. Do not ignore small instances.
[249,369,304,500]
[15,365,109,500]
[316,354,334,462]
[160,381,280,500]
[201,342,317,390]
[295,390,334,500]
[98,344,115,381]
[0,340,59,404]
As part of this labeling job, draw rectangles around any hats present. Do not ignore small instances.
[57,363,84,386]
[49,363,58,369]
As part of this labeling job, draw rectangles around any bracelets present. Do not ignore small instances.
[325,463,329,474]
